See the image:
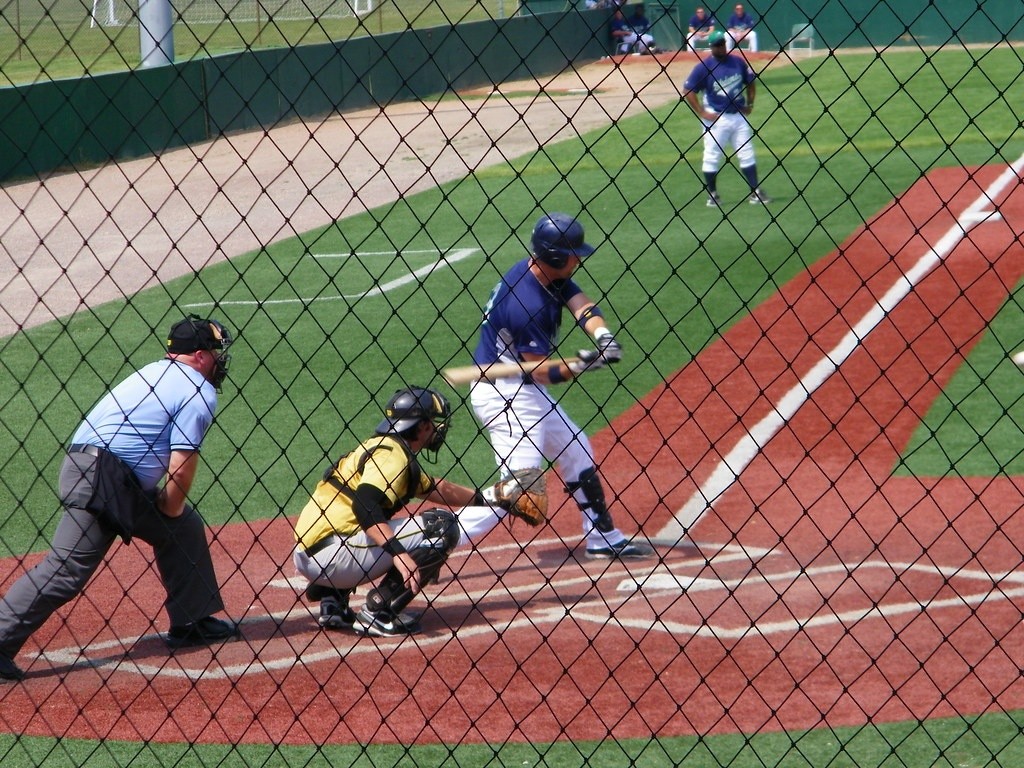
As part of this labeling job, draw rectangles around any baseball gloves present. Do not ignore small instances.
[494,468,549,528]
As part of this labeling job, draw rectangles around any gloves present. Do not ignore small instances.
[592,328,622,363]
[572,350,602,372]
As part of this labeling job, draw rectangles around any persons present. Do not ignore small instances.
[686,7,715,52]
[585,0,627,10]
[452,216,655,560]
[724,3,757,54]
[0,313,239,679]
[612,4,663,55]
[293,383,548,637]
[682,31,773,207]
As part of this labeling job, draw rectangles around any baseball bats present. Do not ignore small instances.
[444,356,579,389]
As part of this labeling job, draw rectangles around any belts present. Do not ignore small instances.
[67,443,100,460]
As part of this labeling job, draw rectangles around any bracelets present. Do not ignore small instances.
[548,364,568,385]
[747,101,754,107]
[474,491,485,506]
[578,306,604,333]
[381,536,407,557]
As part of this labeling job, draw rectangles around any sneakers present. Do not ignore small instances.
[750,189,772,204]
[0,654,24,681]
[707,195,721,207]
[166,617,240,646]
[319,600,356,628]
[352,603,423,637]
[585,540,655,560]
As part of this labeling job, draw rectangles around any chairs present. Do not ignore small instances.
[788,23,814,56]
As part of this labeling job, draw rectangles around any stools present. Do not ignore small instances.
[615,42,636,54]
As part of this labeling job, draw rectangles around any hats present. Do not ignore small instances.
[168,318,228,354]
[376,388,449,434]
[709,30,726,43]
[531,215,596,259]
